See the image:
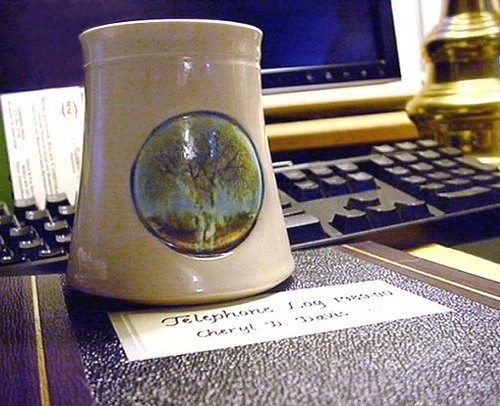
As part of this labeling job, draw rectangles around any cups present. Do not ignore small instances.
[64,19,295,304]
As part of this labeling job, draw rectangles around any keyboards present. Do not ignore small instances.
[0,139,499,274]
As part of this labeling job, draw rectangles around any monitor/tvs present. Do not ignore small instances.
[0,1,421,116]
